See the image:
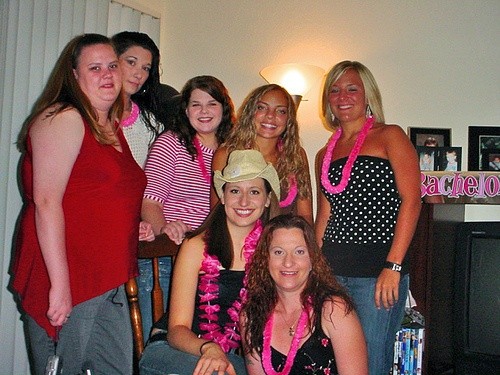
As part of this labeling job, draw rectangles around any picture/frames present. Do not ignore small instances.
[439,147,462,171]
[468,126,500,171]
[481,148,500,171]
[408,127,451,147]
[416,146,438,171]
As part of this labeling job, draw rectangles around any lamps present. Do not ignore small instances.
[260,63,325,108]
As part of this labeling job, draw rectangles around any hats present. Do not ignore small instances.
[214,150,281,204]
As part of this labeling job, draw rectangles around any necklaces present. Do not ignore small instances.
[277,303,301,336]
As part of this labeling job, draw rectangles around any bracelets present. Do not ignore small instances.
[199,341,214,356]
[382,261,402,273]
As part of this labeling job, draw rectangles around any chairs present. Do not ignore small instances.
[125,232,192,361]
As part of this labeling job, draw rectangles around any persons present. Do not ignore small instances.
[314,60,421,374]
[12,34,148,375]
[210,84,313,226]
[139,149,281,375]
[489,156,500,170]
[140,76,236,347]
[443,150,458,171]
[240,214,368,375]
[108,31,164,170]
[421,152,432,170]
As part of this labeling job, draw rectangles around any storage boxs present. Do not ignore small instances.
[433,204,500,222]
